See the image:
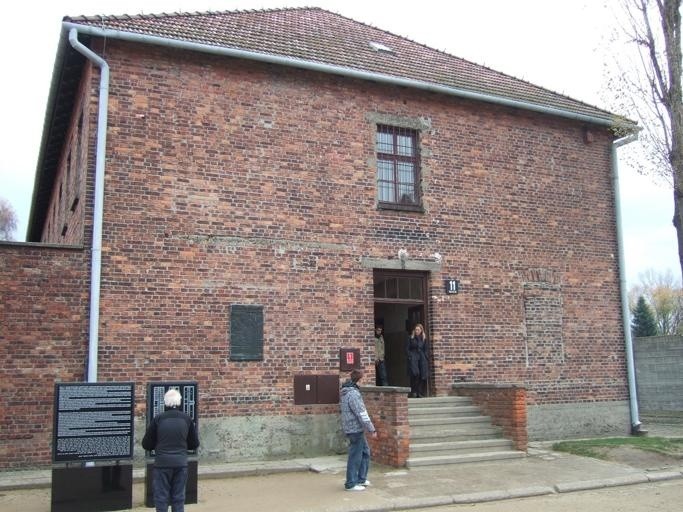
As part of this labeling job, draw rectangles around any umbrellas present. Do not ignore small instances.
[410,390,426,398]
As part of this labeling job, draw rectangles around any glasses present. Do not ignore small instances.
[388,249,410,264]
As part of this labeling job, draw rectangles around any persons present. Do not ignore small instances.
[374,324,388,385]
[139,389,199,511]
[405,323,427,397]
[337,370,378,490]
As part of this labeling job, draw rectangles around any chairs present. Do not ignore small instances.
[345,479,370,491]
